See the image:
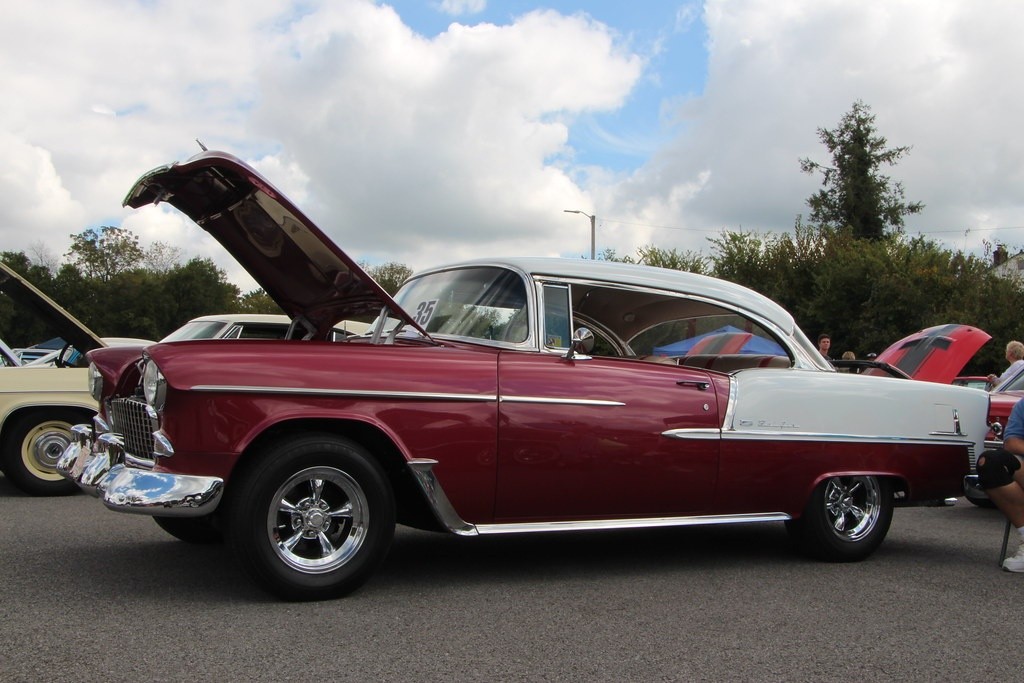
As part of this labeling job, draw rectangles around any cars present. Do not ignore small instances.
[685,324,1024,507]
[0,262,372,496]
[56,138,992,595]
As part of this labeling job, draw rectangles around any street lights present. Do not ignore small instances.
[564,210,595,260]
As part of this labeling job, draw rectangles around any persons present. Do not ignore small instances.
[866,353,877,362]
[985,341,1024,391]
[975,396,1024,574]
[841,351,860,374]
[817,334,833,362]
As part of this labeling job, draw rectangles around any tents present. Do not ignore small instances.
[652,324,787,366]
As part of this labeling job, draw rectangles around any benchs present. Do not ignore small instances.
[637,355,791,372]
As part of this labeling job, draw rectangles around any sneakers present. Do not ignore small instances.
[1001,538,1024,573]
